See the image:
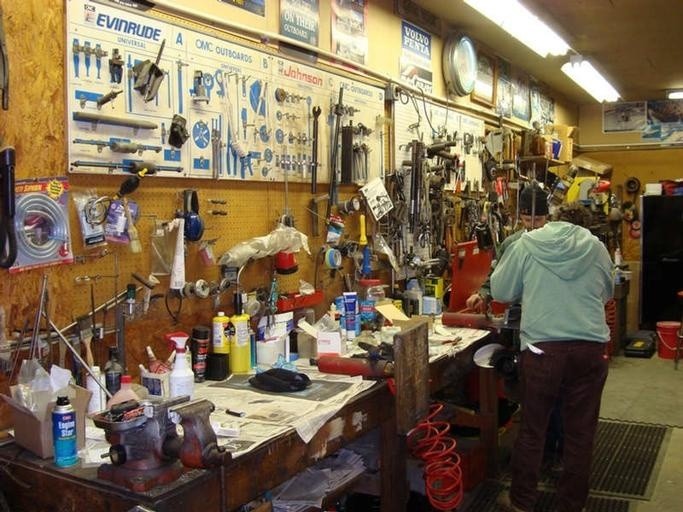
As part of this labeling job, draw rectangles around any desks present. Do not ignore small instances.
[2,327,501,511]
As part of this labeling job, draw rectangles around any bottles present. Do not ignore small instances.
[49,394,79,469]
[84,345,137,416]
[192,310,252,382]
[358,278,385,330]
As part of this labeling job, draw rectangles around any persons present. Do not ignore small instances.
[465,184,562,472]
[489,202,616,510]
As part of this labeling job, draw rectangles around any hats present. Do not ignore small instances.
[520,181,549,216]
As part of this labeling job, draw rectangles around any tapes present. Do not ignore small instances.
[325,248,344,268]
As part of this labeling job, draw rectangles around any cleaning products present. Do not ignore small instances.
[164,331,197,402]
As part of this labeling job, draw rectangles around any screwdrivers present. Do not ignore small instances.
[305,207,342,232]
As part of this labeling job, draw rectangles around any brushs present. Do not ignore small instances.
[121,197,143,253]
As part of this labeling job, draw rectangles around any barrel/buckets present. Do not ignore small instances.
[657,321,680,359]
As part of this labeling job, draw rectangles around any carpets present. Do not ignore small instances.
[454,415,683,511]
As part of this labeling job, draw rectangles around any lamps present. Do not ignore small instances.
[462,0,624,107]
